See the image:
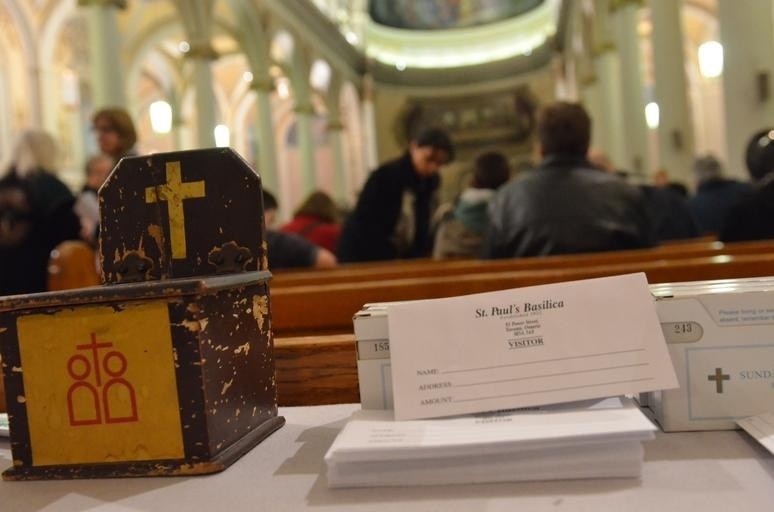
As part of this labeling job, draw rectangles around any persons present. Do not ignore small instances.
[2,106,138,298]
[258,97,773,271]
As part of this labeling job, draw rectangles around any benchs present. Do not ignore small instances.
[270,235,774,406]
